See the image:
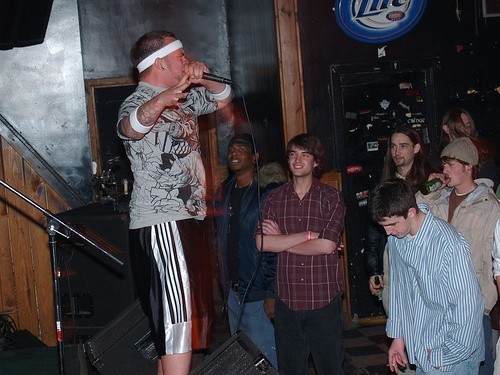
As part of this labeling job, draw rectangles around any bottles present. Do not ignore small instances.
[420,177,446,195]
[374,276,382,301]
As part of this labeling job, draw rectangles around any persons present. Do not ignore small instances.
[117,30,235,375]
[213,105,500,375]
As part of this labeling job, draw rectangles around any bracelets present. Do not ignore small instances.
[129,105,154,134]
[210,83,231,100]
[307,231,312,241]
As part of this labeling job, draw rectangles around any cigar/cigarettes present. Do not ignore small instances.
[387,363,395,366]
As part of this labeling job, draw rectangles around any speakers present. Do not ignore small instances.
[0,0,54,51]
[1,298,279,375]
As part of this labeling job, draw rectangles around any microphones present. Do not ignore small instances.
[202,72,236,86]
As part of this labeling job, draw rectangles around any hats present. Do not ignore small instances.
[440,137,479,166]
